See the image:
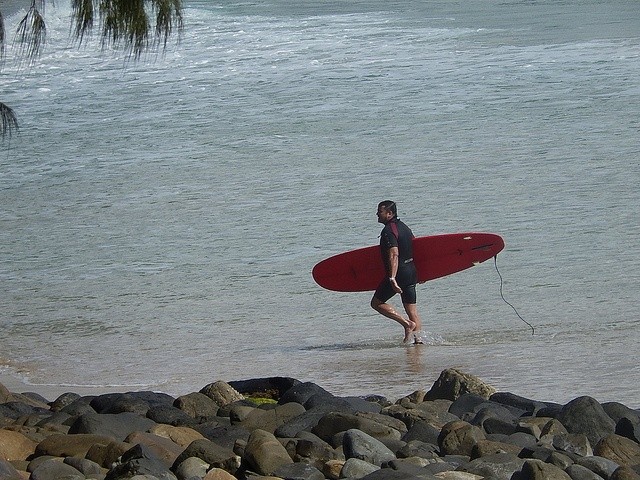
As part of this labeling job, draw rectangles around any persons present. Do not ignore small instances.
[370,200,427,345]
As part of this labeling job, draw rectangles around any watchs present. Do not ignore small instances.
[388,276,397,282]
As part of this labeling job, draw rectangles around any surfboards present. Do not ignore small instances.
[312,231,504,293]
